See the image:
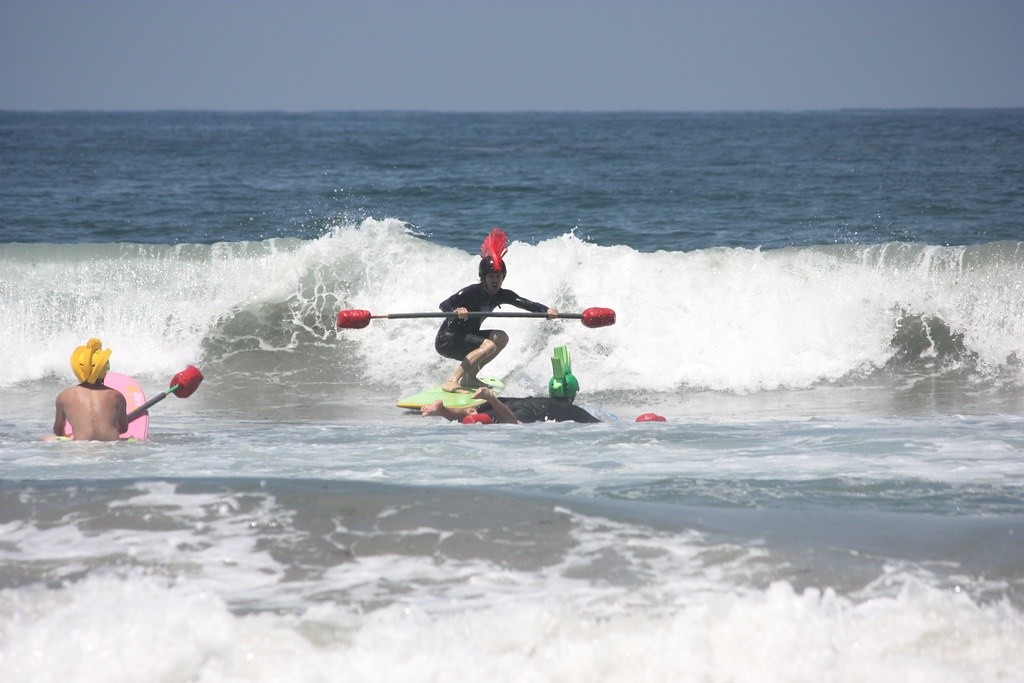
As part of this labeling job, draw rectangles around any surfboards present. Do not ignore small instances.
[396,376,505,410]
[64,370,149,440]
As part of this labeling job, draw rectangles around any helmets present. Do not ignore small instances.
[549,345,580,399]
[71,339,111,384]
[477,228,507,277]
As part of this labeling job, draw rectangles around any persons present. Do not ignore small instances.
[421,386,602,424]
[53,347,129,442]
[435,255,558,394]
